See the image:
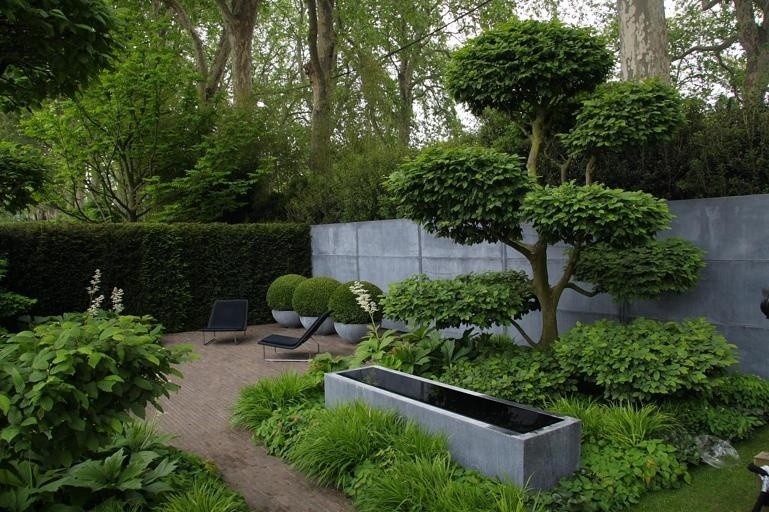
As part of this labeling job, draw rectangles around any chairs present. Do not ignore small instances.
[199,299,335,363]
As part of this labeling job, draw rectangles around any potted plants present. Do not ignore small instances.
[265,273,385,343]
